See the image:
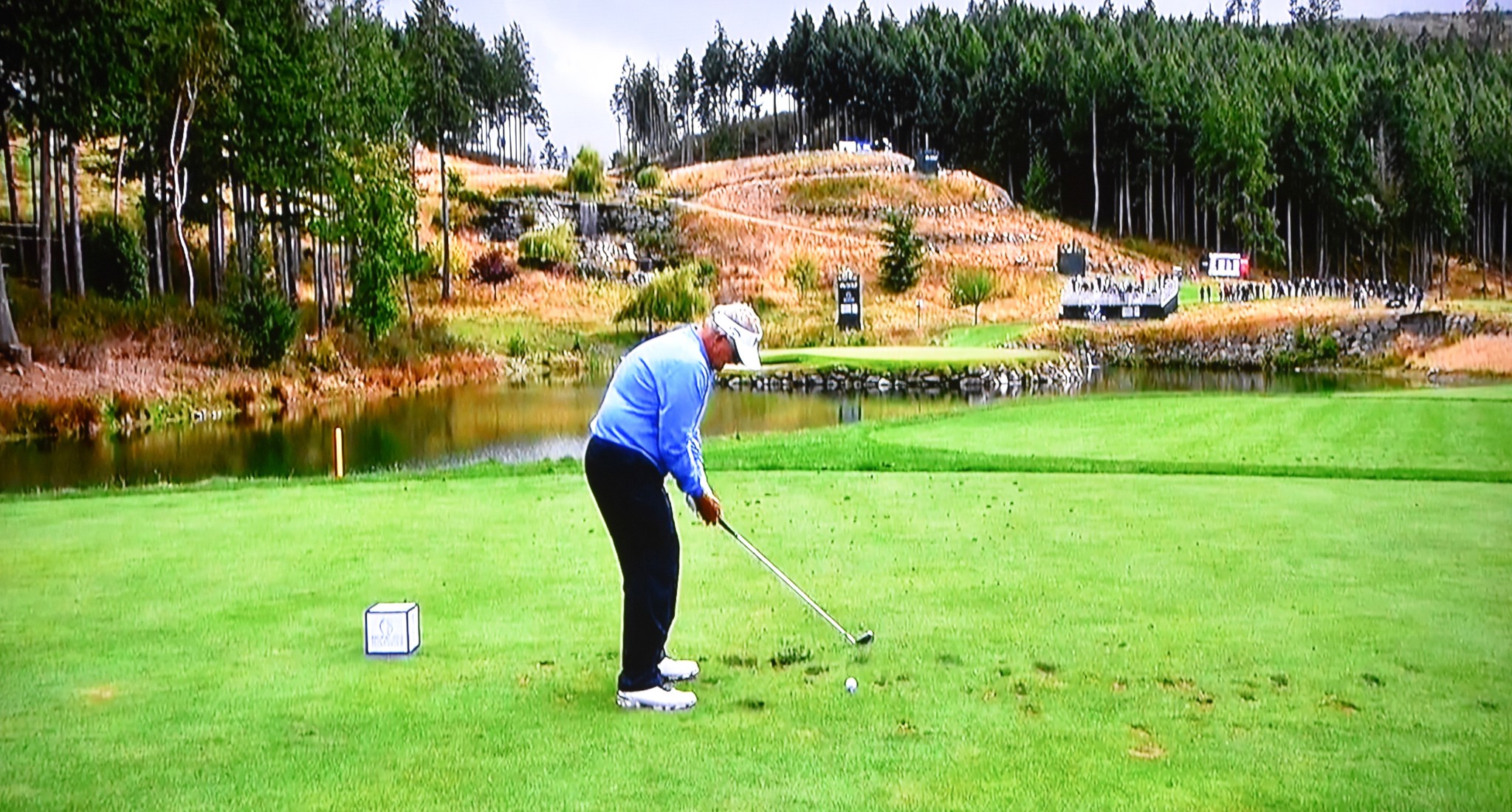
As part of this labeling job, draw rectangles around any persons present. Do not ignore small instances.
[580,301,764,711]
[1068,263,1424,313]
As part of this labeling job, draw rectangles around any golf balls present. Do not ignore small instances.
[846,676,858,689]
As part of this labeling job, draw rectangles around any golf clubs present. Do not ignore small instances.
[686,495,876,648]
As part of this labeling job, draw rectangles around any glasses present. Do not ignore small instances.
[724,337,743,367]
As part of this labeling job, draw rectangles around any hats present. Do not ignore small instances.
[714,301,767,375]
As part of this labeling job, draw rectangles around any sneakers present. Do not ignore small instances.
[614,682,699,714]
[650,650,702,682]
